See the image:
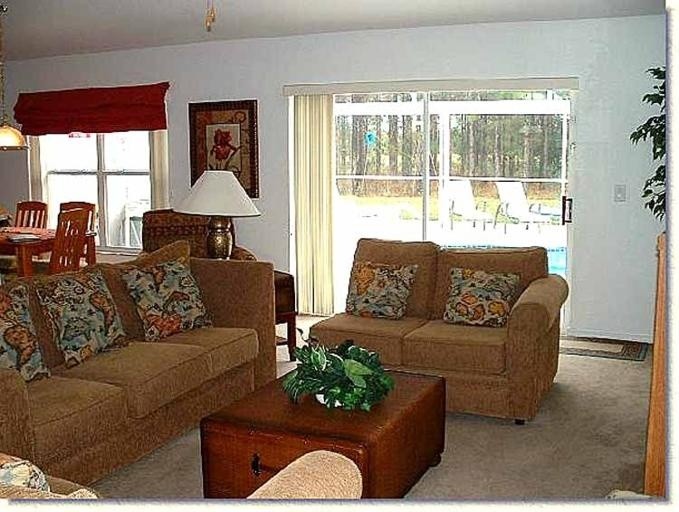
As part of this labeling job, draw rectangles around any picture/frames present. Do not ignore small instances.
[184,97,261,200]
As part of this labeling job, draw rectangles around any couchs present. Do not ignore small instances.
[306,233,573,431]
[0,446,362,498]
[0,250,278,487]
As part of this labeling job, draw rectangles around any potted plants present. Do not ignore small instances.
[276,326,396,415]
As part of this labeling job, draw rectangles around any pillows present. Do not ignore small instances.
[0,452,53,493]
[0,282,51,385]
[438,265,522,332]
[115,254,214,343]
[341,259,420,321]
[32,269,130,372]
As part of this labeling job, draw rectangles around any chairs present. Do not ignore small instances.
[135,208,301,366]
[437,170,561,233]
[11,200,49,261]
[55,200,97,267]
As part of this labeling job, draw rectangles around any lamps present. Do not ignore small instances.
[172,171,264,259]
[0,4,31,152]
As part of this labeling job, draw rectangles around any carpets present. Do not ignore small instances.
[560,335,650,363]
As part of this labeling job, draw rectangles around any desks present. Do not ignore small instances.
[0,226,96,277]
